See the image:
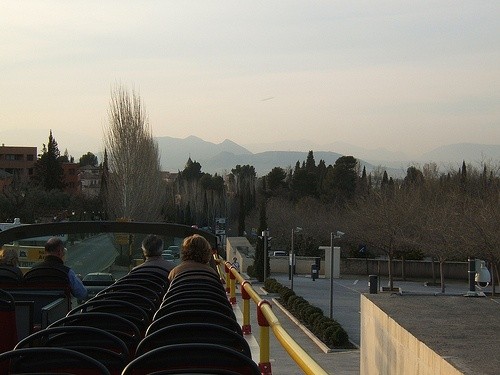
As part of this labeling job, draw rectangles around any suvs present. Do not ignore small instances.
[168,245,181,259]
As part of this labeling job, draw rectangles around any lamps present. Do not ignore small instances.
[476,259,491,296]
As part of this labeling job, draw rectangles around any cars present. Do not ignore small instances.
[162,253,176,267]
[76,271,118,305]
[162,249,175,259]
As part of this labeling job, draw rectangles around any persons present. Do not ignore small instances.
[168,234,218,281]
[0,246,23,277]
[132,234,176,271]
[29,237,87,300]
[231,257,240,289]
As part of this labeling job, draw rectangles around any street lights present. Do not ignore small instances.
[71,212,105,239]
[329,230,345,320]
[290,227,302,290]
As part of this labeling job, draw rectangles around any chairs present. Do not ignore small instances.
[0,266,261,375]
